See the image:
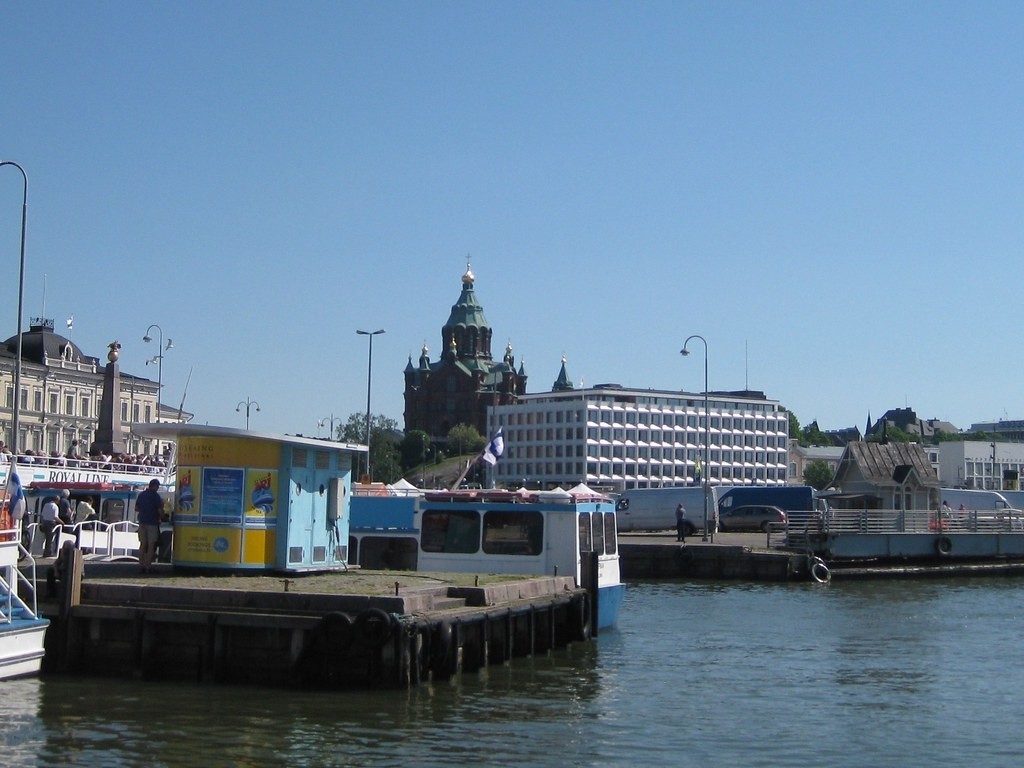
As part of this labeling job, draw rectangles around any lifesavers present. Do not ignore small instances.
[935,536,954,557]
[811,563,831,584]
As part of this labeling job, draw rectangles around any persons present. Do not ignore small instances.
[75,495,96,550]
[675,504,686,542]
[941,500,965,532]
[42,489,73,558]
[0,440,176,477]
[135,479,165,573]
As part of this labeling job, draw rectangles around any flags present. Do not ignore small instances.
[482,428,504,465]
[7,461,26,519]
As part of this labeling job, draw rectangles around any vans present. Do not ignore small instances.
[715,486,818,522]
[610,487,718,532]
[940,488,1014,515]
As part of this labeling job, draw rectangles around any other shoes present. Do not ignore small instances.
[43,553,54,557]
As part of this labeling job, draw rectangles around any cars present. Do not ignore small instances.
[716,505,790,533]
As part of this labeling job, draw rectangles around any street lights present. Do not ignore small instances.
[145,325,174,422]
[236,396,260,430]
[321,414,342,442]
[355,329,387,478]
[681,334,711,541]
